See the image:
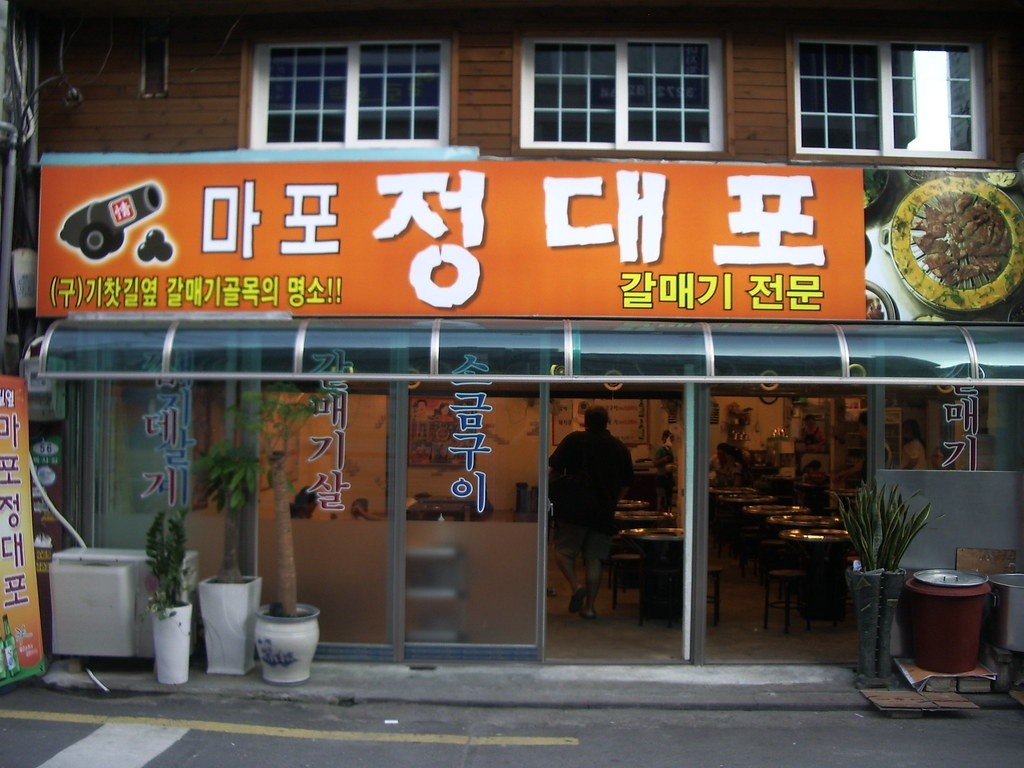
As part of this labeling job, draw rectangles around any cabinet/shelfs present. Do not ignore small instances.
[845,408,903,450]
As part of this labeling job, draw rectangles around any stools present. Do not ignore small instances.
[610,554,640,610]
[716,513,737,559]
[638,569,683,629]
[758,540,787,583]
[707,568,722,628]
[764,569,813,635]
[738,526,761,578]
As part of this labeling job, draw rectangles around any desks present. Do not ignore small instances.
[405,486,852,633]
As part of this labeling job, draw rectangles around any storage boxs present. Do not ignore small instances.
[767,436,795,454]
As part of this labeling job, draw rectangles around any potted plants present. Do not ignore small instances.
[223,382,351,686]
[189,437,266,677]
[145,506,197,685]
[827,479,945,689]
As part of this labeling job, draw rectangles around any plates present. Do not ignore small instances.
[878,175,1024,317]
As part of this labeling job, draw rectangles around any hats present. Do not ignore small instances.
[803,415,816,420]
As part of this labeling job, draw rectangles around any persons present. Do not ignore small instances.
[547,406,634,619]
[290,487,316,518]
[798,415,826,454]
[895,419,928,470]
[832,411,892,489]
[709,443,747,488]
[932,447,953,470]
[652,430,674,512]
[351,499,386,521]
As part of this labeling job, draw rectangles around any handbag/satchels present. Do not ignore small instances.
[548,430,581,507]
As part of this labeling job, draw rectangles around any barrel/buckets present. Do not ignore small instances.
[983,573,1024,652]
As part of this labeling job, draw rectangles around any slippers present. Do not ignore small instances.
[580,607,597,619]
[569,586,585,613]
